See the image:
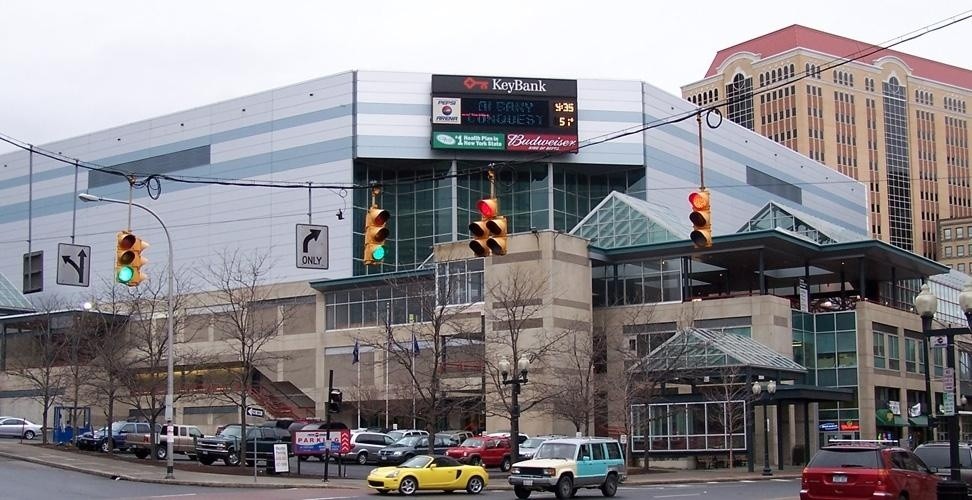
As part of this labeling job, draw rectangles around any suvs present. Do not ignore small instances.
[386,430,431,445]
[508,436,627,499]
[445,436,511,470]
[330,432,397,465]
[197,426,293,466]
[287,420,321,432]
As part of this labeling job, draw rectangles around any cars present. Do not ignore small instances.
[83,421,165,453]
[799,439,942,499]
[910,441,972,500]
[517,437,556,458]
[0,416,43,440]
[375,435,455,466]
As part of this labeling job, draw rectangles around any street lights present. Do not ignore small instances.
[78,193,175,478]
[914,284,972,481]
[496,354,530,466]
[751,380,775,476]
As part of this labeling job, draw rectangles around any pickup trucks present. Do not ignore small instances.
[125,424,214,461]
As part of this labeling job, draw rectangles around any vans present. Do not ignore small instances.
[294,422,350,461]
[436,430,474,446]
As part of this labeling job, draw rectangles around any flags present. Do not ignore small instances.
[352,335,359,365]
[411,333,420,358]
[385,309,392,338]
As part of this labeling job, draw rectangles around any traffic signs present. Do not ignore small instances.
[246,405,266,481]
[297,224,330,270]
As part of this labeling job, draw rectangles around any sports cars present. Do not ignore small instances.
[367,454,489,496]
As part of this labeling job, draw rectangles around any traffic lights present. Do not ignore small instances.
[367,209,387,262]
[687,191,713,248]
[329,390,342,411]
[140,239,149,284]
[117,233,143,284]
[468,199,508,256]
